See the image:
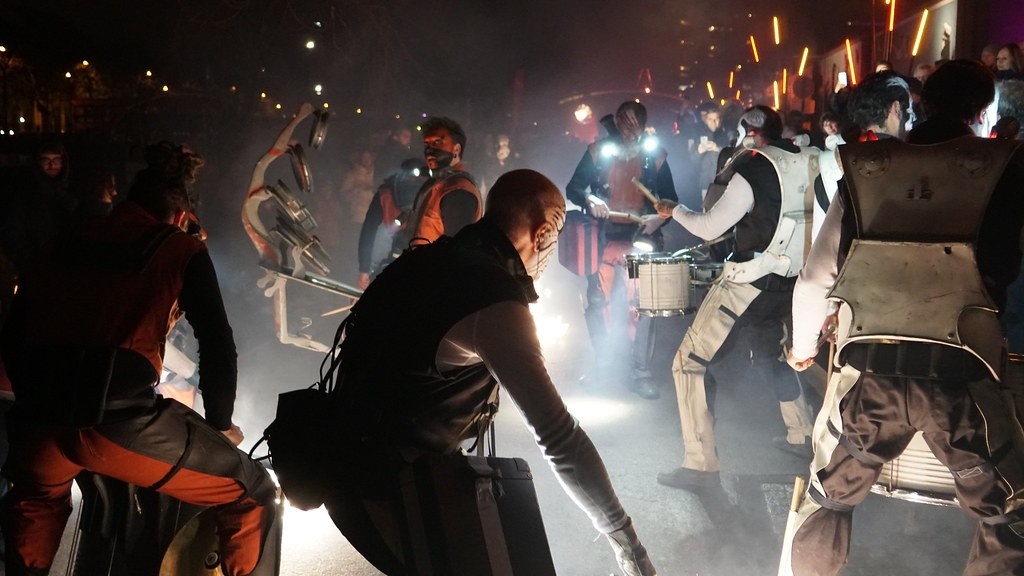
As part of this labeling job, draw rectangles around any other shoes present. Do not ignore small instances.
[658,466,720,488]
[628,377,660,398]
[773,435,813,459]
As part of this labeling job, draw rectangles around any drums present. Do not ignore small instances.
[241,102,332,280]
[625,252,736,319]
[870,301,999,510]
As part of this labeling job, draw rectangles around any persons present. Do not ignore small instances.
[1,34,1023,576]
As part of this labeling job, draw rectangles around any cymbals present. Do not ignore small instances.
[159,502,235,575]
